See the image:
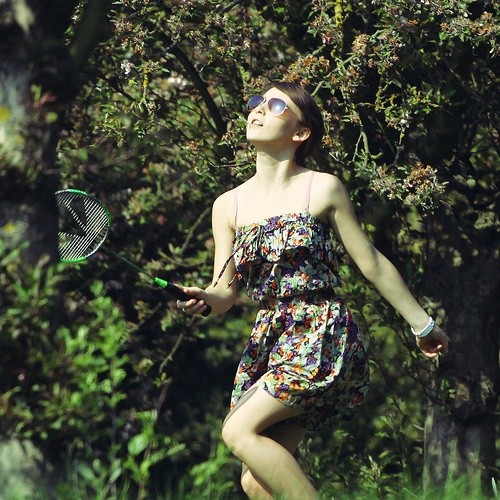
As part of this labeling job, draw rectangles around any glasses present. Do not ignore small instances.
[246,95,302,127]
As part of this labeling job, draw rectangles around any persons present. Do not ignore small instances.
[171,81,450,500]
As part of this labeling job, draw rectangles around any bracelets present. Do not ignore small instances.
[411,316,435,339]
